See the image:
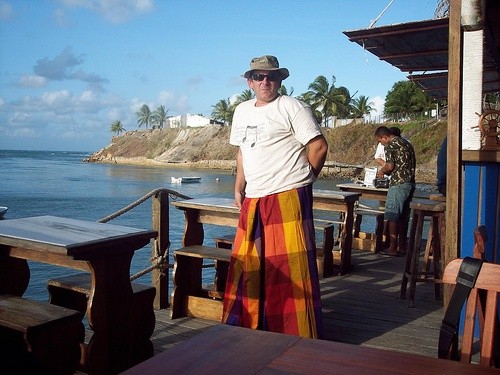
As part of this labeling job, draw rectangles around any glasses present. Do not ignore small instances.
[249,73,280,82]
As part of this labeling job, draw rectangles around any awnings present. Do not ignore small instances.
[342,16,449,72]
[406,72,448,99]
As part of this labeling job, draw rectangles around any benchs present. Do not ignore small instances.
[0,295,83,375]
[45,273,157,364]
[172,209,384,319]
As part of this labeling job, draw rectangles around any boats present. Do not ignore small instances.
[170,176,201,184]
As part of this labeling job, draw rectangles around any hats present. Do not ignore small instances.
[244,55,289,81]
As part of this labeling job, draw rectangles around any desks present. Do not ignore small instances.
[170,198,242,322]
[118,324,500,375]
[336,183,390,256]
[0,215,158,375]
[312,187,363,276]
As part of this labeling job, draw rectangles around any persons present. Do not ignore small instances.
[374,126,416,257]
[222,55,328,339]
[436,136,447,196]
[374,127,414,167]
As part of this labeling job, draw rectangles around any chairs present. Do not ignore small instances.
[440,258,500,366]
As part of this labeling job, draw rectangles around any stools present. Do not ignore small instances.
[401,201,446,307]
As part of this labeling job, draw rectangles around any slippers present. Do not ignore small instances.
[397,250,406,257]
[379,248,397,256]
